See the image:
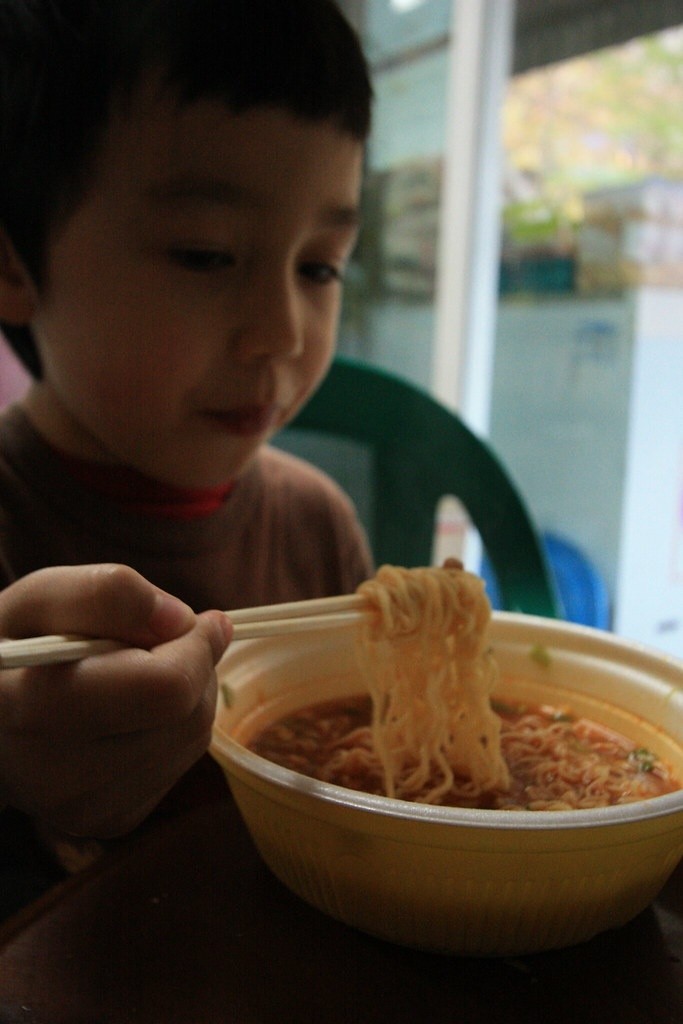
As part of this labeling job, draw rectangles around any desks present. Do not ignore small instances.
[0,790,683,1024]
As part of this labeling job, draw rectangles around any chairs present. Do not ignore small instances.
[290,359,555,621]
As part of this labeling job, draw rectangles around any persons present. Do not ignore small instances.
[0,3,463,893]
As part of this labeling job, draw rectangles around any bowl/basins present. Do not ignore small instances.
[206,609,683,957]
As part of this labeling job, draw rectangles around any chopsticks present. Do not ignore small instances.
[0,594,382,667]
